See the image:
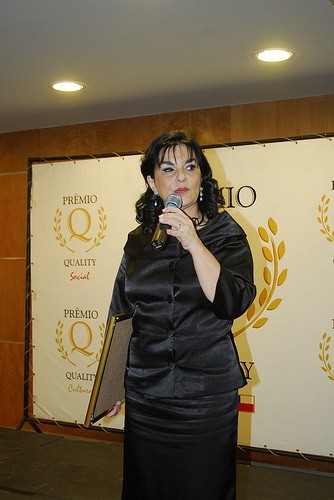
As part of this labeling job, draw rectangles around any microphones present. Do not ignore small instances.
[151,193,181,249]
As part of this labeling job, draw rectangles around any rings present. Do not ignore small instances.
[175,223,181,229]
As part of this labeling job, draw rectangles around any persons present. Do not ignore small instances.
[100,128,259,500]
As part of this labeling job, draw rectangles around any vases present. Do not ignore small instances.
[83,311,137,429]
